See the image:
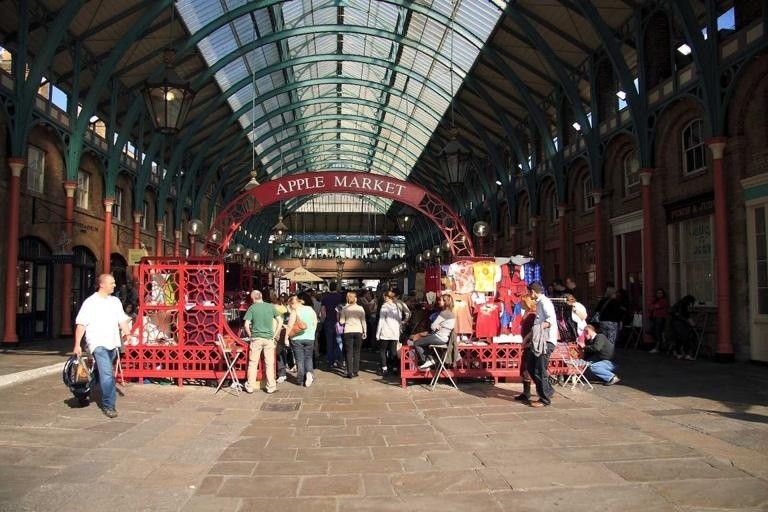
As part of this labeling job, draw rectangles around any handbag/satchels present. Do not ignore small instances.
[288,320,307,337]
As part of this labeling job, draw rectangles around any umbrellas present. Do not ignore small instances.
[281,267,323,282]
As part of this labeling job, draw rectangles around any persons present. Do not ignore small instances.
[243,290,283,393]
[549,282,623,386]
[648,290,694,360]
[514,283,558,405]
[74,273,134,418]
[285,282,412,386]
[414,294,454,369]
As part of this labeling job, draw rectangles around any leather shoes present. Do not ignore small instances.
[531,400,545,407]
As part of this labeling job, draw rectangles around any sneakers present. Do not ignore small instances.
[377,369,398,376]
[649,348,695,361]
[348,372,358,378]
[73,391,90,406]
[604,376,620,386]
[276,376,287,383]
[420,359,436,369]
[102,407,118,417]
[245,381,253,393]
[305,372,312,386]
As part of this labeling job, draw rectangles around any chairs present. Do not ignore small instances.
[89,345,126,397]
[214,334,244,396]
[428,329,459,391]
[559,342,593,390]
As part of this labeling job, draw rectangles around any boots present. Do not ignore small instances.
[515,381,531,399]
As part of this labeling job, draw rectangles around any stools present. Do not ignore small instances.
[624,324,643,350]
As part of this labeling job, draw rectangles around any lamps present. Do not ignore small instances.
[456,232,472,244]
[439,15,477,176]
[299,214,381,258]
[235,244,245,257]
[415,254,426,264]
[432,245,444,258]
[395,150,417,233]
[244,249,285,273]
[378,204,393,253]
[138,3,196,134]
[271,123,287,236]
[391,262,409,272]
[187,218,203,237]
[442,239,454,251]
[472,220,488,237]
[290,172,301,251]
[225,235,235,250]
[245,57,260,191]
[207,230,223,243]
[422,250,433,260]
